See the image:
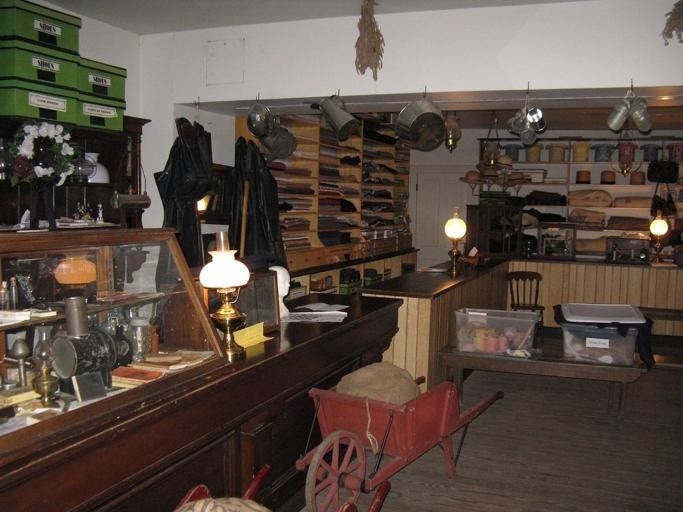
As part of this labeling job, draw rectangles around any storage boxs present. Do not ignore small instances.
[0,1,129,134]
[551,301,648,367]
[453,305,540,361]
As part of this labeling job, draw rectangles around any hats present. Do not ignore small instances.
[459,170,482,184]
[479,166,498,178]
[501,172,532,187]
[495,154,513,169]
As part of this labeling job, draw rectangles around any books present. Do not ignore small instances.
[131,357,184,371]
[158,345,177,355]
[179,353,202,365]
[142,350,181,363]
[0,385,39,410]
[308,285,337,294]
[111,365,164,385]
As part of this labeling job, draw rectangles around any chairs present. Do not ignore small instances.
[506,271,544,342]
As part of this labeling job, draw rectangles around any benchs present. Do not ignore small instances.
[435,338,648,422]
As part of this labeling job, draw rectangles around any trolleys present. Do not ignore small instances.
[171,463,392,512]
[295,375,505,511]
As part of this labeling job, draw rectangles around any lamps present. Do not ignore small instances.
[645,209,678,268]
[443,204,468,279]
[198,228,250,364]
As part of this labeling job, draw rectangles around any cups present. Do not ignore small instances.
[506,106,547,147]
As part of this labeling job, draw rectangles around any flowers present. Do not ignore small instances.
[1,119,80,188]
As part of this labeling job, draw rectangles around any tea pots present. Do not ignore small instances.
[310,95,360,142]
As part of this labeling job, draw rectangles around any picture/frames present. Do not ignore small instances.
[235,269,280,336]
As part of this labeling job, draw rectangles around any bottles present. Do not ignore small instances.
[607,94,653,134]
[0,280,10,311]
[127,317,152,360]
[10,276,19,311]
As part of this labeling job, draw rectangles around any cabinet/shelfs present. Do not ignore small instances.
[0,229,229,461]
[231,112,421,303]
[472,134,682,229]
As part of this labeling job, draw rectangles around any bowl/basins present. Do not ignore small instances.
[396,97,449,153]
[246,103,275,138]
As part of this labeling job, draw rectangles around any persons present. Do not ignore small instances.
[269,265,292,318]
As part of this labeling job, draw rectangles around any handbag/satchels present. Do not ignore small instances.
[650,183,677,216]
[646,158,678,183]
[483,115,500,165]
